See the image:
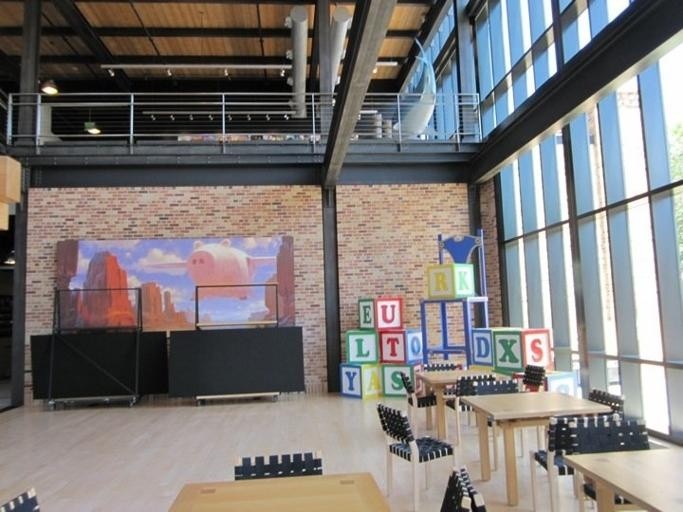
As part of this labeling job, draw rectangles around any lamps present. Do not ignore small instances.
[40,79,58,94]
[86,110,101,134]
[100,62,397,78]
[143,111,296,122]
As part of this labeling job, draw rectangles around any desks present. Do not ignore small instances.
[416,373,514,441]
[459,391,613,507]
[562,447,682,511]
[170,470,393,511]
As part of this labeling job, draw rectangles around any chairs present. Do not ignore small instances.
[568,418,652,512]
[377,402,457,510]
[424,363,456,371]
[0,487,40,512]
[528,413,621,511]
[444,374,497,444]
[400,372,450,438]
[233,451,323,480]
[588,389,627,421]
[471,379,526,471]
[523,364,546,391]
[436,466,472,511]
[461,464,487,511]
